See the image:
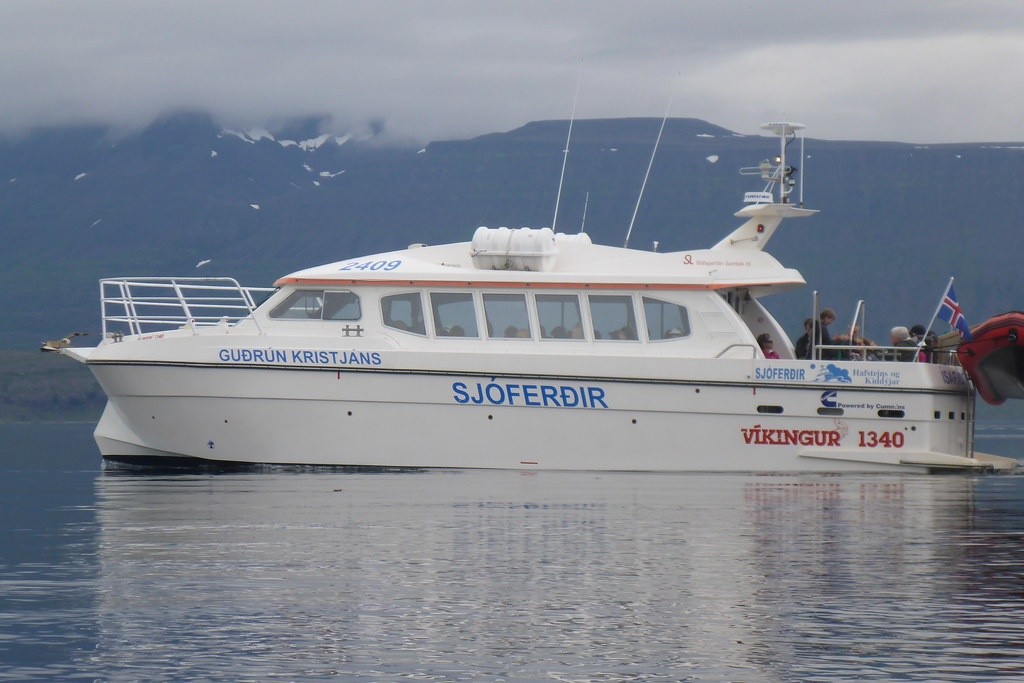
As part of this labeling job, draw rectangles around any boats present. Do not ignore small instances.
[35,74,1023,475]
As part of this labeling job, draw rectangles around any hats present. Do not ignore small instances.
[909,325,926,338]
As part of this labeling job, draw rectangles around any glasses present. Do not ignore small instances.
[764,341,773,343]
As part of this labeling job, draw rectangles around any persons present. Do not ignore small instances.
[796,309,877,360]
[757,334,780,360]
[885,325,938,364]
[393,320,684,340]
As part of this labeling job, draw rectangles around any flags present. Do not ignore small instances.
[938,288,973,342]
